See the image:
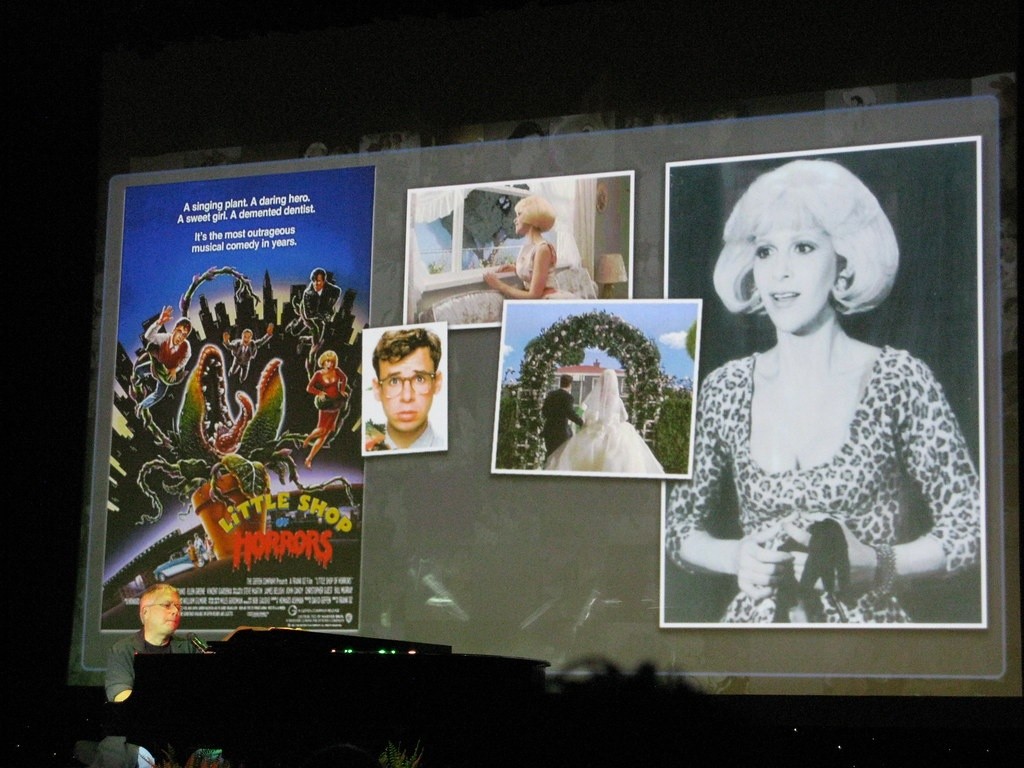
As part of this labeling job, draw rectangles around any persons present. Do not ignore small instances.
[133,267,348,471]
[366,328,442,450]
[105,583,206,702]
[539,368,665,472]
[484,195,579,299]
[666,158,980,622]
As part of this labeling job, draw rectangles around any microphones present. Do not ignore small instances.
[186,632,207,653]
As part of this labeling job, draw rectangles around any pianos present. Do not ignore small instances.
[77,631,552,768]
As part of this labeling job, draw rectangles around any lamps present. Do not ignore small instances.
[593,253,629,302]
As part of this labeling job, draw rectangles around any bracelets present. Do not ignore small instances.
[873,543,896,600]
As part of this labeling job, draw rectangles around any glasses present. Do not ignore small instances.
[376,369,439,400]
[140,601,181,611]
